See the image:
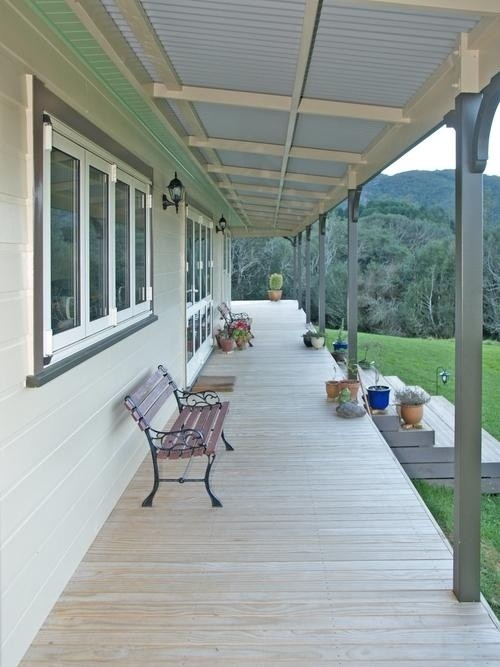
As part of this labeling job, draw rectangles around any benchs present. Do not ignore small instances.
[122,363,235,510]
[217,301,254,349]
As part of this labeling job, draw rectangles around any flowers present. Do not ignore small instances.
[218,321,253,351]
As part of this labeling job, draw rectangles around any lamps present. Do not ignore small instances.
[435,365,449,396]
[161,168,185,215]
[215,212,227,236]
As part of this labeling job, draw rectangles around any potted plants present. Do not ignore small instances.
[395,386,431,426]
[312,334,328,347]
[357,341,382,371]
[367,367,391,412]
[267,271,286,302]
[325,364,340,403]
[303,330,313,346]
[332,329,348,350]
[330,349,345,362]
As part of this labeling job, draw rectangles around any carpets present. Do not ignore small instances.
[190,374,236,395]
